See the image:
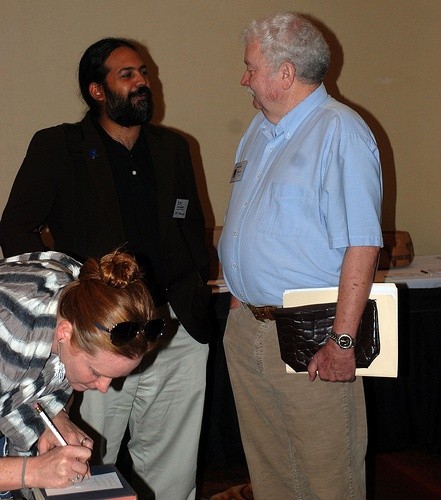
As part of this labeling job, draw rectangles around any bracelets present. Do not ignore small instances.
[21,456,33,491]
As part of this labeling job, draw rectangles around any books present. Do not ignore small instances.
[281,282,398,377]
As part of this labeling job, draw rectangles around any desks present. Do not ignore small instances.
[198,254,441,478]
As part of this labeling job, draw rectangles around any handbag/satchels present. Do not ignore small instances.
[275,298,379,372]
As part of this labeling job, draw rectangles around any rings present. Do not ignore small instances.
[72,475,80,481]
[79,438,89,446]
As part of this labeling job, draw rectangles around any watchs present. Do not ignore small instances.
[330,332,353,350]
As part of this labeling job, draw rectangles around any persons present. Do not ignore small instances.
[0,36,215,500]
[216,12,383,500]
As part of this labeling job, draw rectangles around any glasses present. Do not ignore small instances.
[91,319,167,346]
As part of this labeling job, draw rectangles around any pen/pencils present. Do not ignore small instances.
[35,404,91,480]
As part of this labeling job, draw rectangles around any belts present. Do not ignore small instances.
[240,301,278,322]
